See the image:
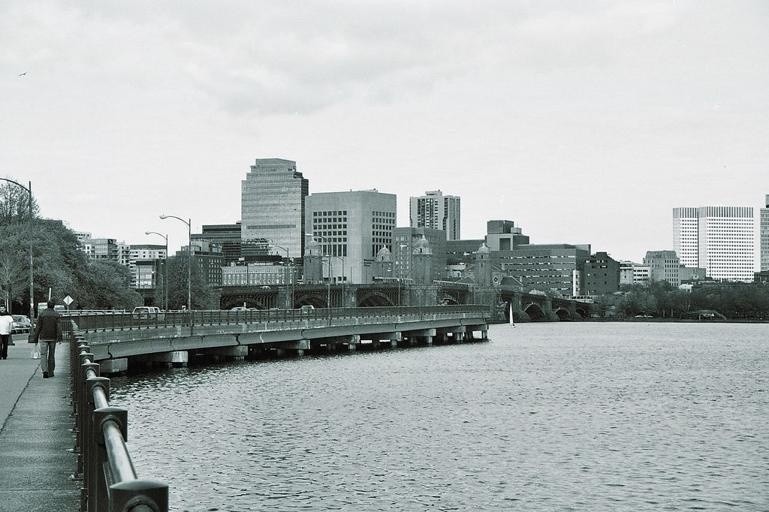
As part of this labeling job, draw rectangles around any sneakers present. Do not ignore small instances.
[43,371,55,378]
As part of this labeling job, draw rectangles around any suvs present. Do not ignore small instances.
[131,306,166,325]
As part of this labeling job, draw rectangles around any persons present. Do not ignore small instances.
[181,305,186,327]
[34,298,62,378]
[0,304,14,360]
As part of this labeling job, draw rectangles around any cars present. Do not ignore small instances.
[9,312,38,335]
[229,304,315,312]
[633,314,654,318]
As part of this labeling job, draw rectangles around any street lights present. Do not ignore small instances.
[145,231,169,312]
[305,232,332,308]
[267,243,290,310]
[399,244,423,306]
[0,176,38,344]
[159,214,192,310]
